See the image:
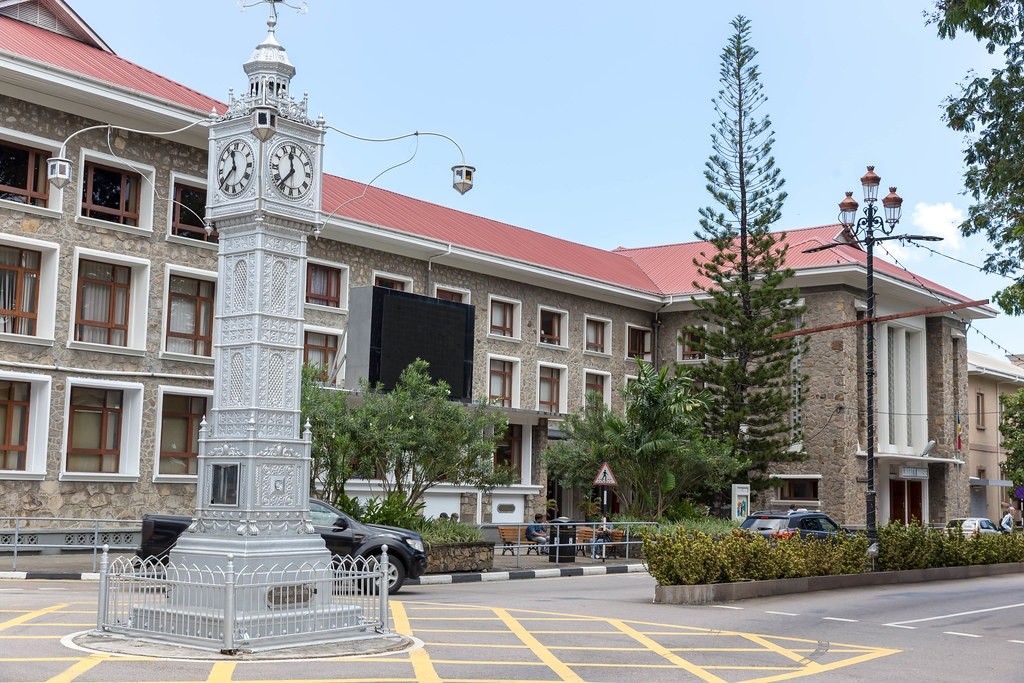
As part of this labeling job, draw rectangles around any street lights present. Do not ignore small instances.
[836,165,904,572]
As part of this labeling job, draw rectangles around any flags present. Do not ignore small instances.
[957,416,963,453]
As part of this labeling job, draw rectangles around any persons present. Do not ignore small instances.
[590,514,612,559]
[440,512,459,522]
[528,513,551,555]
[1001,507,1017,533]
[788,503,797,511]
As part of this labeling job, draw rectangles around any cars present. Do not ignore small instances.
[942,517,1003,542]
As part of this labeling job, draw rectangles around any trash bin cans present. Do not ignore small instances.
[549,517,576,563]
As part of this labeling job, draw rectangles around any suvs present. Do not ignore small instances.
[738,508,858,549]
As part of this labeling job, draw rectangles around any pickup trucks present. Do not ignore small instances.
[132,497,428,596]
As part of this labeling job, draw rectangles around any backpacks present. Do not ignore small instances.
[526,521,536,540]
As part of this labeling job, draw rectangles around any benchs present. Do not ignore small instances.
[498,525,540,556]
[574,527,625,559]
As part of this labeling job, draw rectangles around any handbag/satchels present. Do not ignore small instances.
[999,516,1009,530]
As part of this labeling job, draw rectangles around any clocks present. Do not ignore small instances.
[217,138,256,197]
[268,140,314,204]
